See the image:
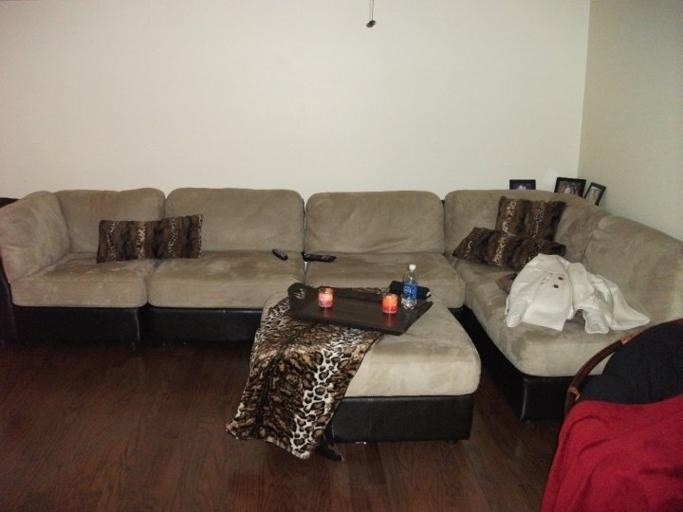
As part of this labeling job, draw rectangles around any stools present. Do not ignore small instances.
[260,283,483,445]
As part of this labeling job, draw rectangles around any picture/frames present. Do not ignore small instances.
[508,177,604,207]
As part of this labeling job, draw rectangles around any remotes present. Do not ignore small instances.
[303,254,336,262]
[272,249,288,260]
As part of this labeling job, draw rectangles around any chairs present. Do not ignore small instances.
[560,314,683,512]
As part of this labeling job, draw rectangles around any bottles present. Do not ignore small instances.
[400,264,417,309]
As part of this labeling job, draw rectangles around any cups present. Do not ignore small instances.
[317,288,333,307]
[382,293,397,314]
